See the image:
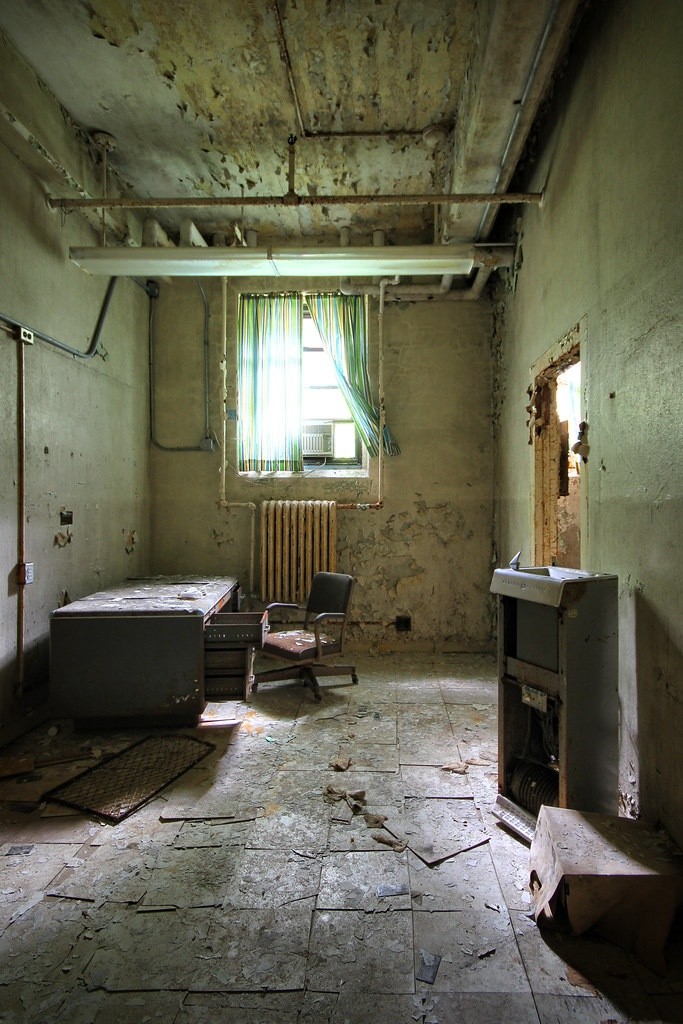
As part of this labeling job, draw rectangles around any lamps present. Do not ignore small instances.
[67,123,476,276]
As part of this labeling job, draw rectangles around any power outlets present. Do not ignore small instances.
[18,562,34,586]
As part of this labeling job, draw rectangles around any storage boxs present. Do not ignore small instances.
[527,805,683,959]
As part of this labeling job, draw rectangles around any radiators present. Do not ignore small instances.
[261,499,335,604]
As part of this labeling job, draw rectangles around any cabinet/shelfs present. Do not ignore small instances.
[488,567,619,842]
[45,575,270,726]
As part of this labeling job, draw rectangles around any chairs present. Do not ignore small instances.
[247,571,359,701]
